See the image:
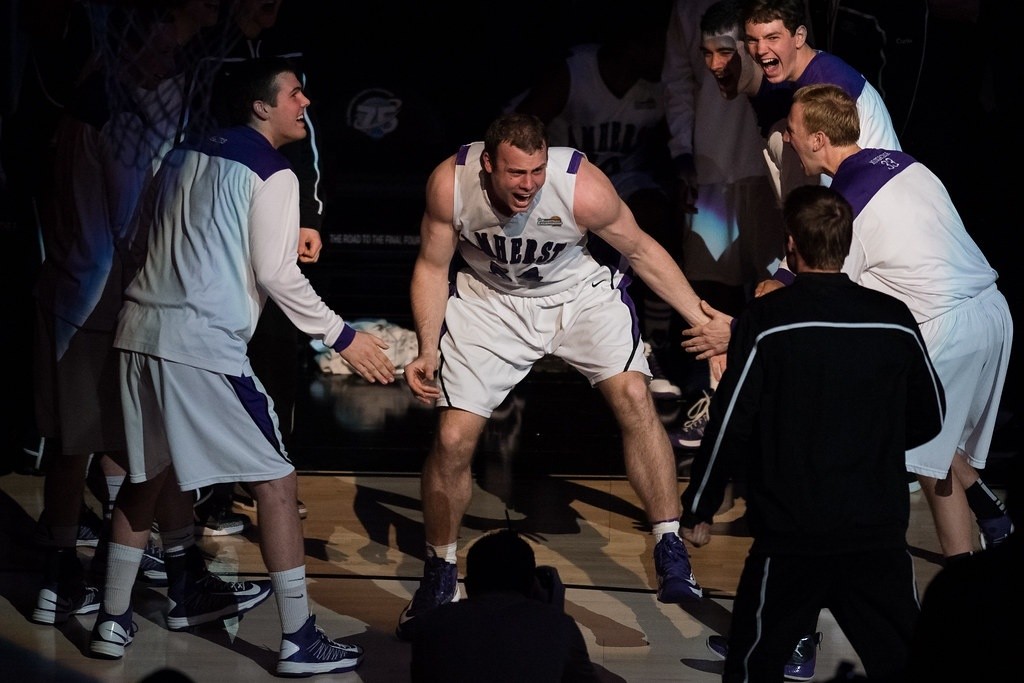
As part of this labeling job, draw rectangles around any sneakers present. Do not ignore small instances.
[276,614,365,677]
[39,507,103,546]
[192,501,250,536]
[31,586,100,624]
[395,546,457,639]
[706,632,823,680]
[233,481,308,517]
[167,571,274,630]
[89,601,138,657]
[646,368,680,397]
[669,387,714,447]
[659,400,679,422]
[140,541,167,579]
[653,532,703,603]
[108,500,161,539]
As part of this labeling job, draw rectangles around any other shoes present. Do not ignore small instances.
[977,516,1015,546]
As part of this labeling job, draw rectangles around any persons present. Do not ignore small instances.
[496,0,1024,683]
[398,104,710,632]
[0,0,403,677]
[408,530,600,683]
